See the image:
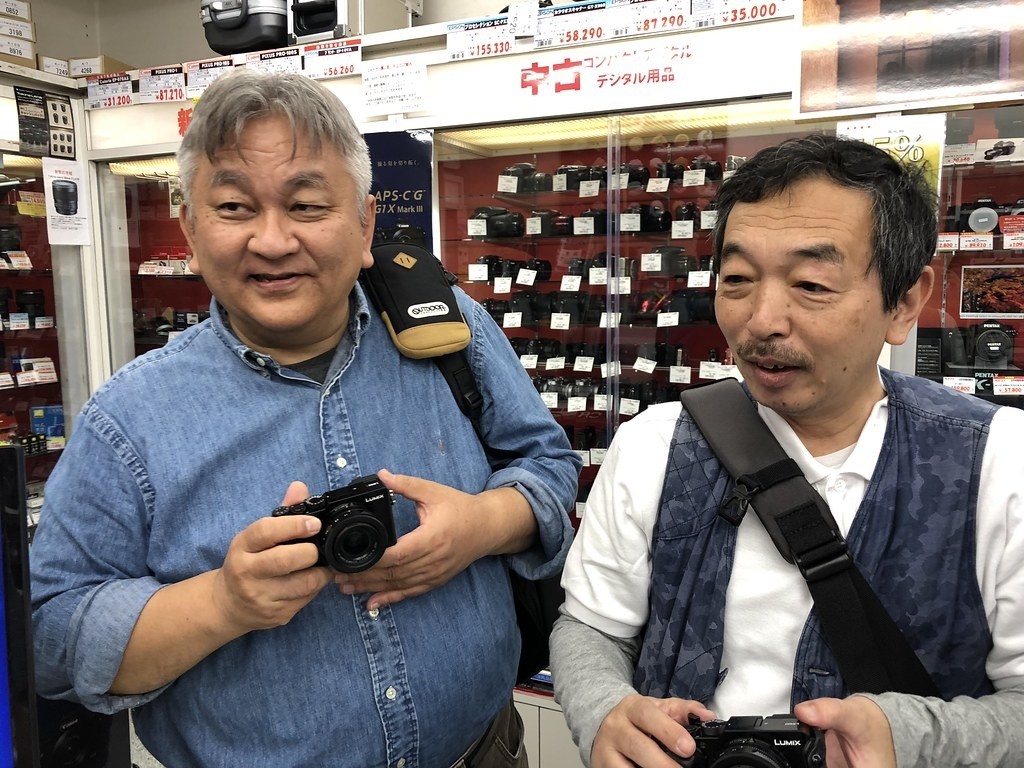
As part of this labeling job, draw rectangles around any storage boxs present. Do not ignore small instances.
[0,0,136,79]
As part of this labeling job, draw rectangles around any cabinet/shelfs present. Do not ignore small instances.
[0,93,1024,532]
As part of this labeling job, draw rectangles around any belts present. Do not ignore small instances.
[448,710,497,768]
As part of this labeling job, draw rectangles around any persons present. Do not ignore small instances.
[540,132,1024,768]
[23,72,582,768]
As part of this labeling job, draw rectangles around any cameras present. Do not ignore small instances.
[945,198,1010,232]
[948,318,1016,367]
[470,158,726,451]
[652,713,828,768]
[271,473,398,572]
